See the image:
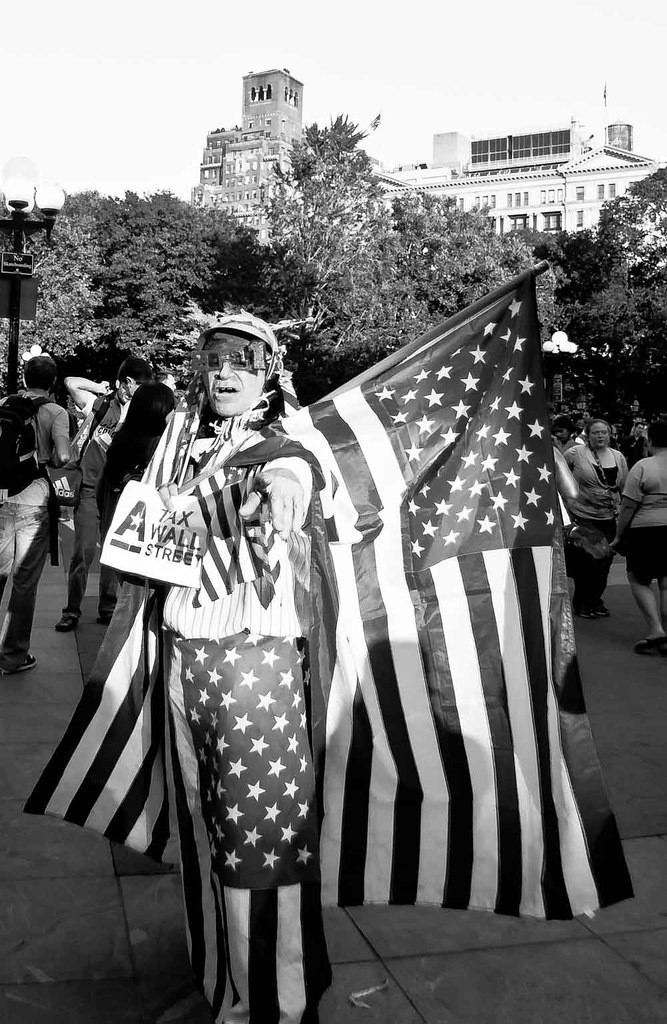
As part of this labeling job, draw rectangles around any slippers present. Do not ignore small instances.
[634,636,666,658]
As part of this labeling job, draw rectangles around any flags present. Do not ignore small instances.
[20,262,635,923]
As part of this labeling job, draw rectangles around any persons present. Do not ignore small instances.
[0,355,176,675]
[141,313,329,1024]
[548,399,667,659]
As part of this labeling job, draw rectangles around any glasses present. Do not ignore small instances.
[191,341,268,374]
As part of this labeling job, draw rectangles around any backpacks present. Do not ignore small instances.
[0,394,58,498]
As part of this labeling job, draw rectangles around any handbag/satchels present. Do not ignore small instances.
[45,463,82,508]
[561,521,616,578]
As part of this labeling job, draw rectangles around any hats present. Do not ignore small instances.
[198,314,279,357]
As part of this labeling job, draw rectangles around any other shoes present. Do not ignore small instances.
[574,604,611,621]
[1,650,36,674]
[96,609,113,624]
[54,615,80,632]
